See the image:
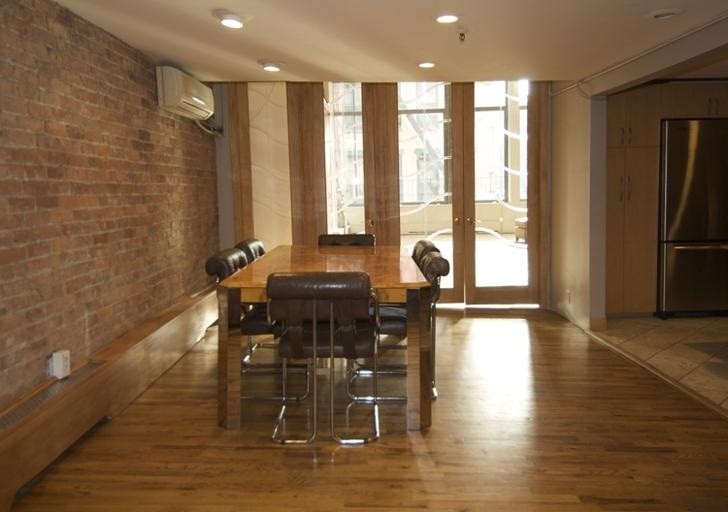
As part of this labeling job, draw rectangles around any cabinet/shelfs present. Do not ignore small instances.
[606,80,728,317]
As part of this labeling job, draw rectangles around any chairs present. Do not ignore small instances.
[348,240,450,404]
[317,234,376,247]
[205,238,311,400]
[265,271,381,444]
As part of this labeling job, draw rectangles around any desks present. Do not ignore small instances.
[218,245,433,430]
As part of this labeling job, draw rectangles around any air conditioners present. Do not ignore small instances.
[156,64,215,122]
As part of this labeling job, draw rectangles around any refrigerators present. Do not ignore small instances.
[659,116,728,319]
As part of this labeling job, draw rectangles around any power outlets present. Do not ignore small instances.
[48,350,73,380]
